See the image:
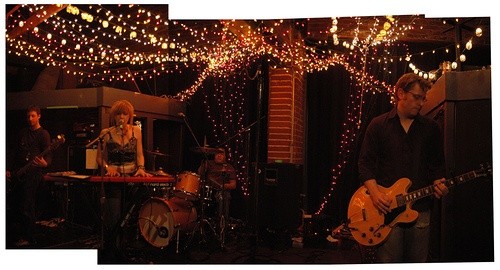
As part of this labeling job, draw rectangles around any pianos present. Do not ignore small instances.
[44,171,176,187]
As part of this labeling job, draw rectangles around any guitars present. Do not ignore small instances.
[346,161,492,247]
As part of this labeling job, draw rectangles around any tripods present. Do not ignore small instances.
[197,149,220,244]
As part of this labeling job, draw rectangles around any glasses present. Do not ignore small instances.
[406,90,428,102]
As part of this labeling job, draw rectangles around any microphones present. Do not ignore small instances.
[119,119,124,129]
[177,112,186,118]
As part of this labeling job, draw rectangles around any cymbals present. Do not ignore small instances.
[144,149,170,156]
[190,146,224,154]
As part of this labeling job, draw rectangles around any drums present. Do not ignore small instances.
[138,171,219,248]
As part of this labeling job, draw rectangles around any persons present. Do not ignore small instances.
[199,148,236,226]
[360,73,448,263]
[96,100,153,178]
[16,103,51,245]
